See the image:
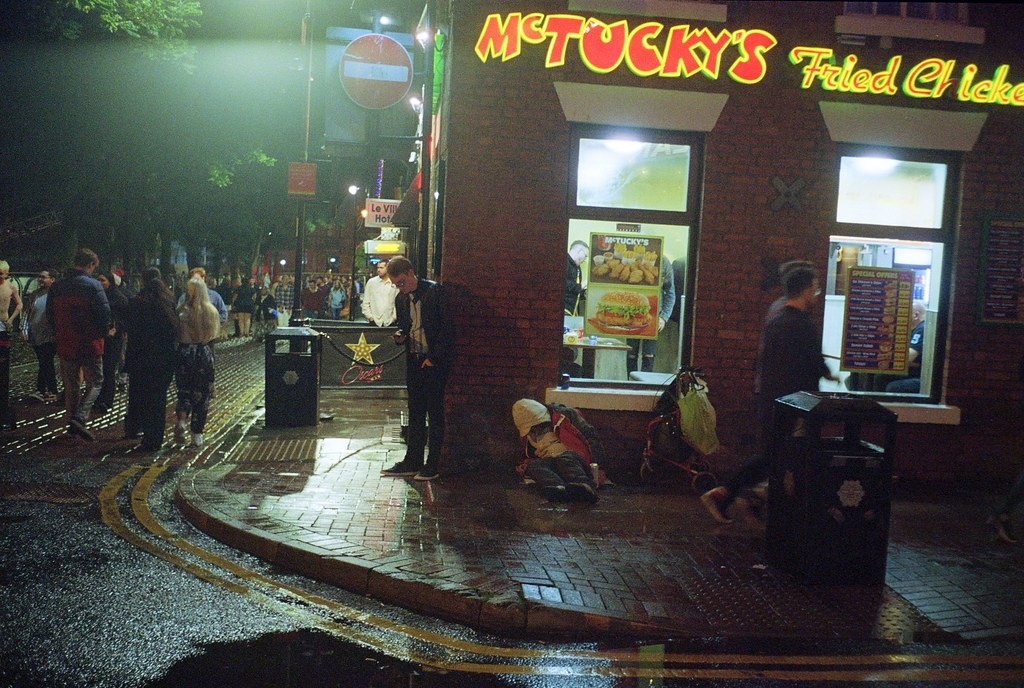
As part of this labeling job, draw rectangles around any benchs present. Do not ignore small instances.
[629,372,677,381]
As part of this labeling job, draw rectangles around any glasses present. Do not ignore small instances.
[392,280,404,288]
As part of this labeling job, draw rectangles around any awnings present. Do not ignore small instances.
[391,171,420,227]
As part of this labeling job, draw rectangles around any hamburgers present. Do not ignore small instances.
[594,291,652,330]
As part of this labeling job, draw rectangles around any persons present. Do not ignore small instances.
[559,240,588,345]
[699,260,842,525]
[511,398,609,506]
[616,223,675,378]
[873,299,924,392]
[380,256,452,480]
[180,268,374,338]
[362,260,400,327]
[0,248,228,453]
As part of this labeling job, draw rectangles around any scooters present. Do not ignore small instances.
[639,366,744,493]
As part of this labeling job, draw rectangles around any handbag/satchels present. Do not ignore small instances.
[677,376,719,454]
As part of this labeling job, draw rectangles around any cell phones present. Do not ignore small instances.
[393,334,401,339]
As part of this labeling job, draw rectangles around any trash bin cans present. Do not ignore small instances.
[266,328,321,429]
[765,391,898,586]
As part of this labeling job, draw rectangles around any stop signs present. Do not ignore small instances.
[337,32,415,110]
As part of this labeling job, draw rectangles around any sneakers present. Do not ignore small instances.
[380,458,426,476]
[412,464,439,480]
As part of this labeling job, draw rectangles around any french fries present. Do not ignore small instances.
[614,243,657,261]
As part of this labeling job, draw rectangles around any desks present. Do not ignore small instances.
[562,337,633,379]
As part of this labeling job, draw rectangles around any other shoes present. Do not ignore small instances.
[542,485,568,503]
[570,481,599,503]
[700,489,736,523]
[45,392,59,402]
[174,418,186,445]
[122,428,145,439]
[69,418,94,440]
[191,433,203,447]
[91,406,108,414]
[30,390,45,401]
